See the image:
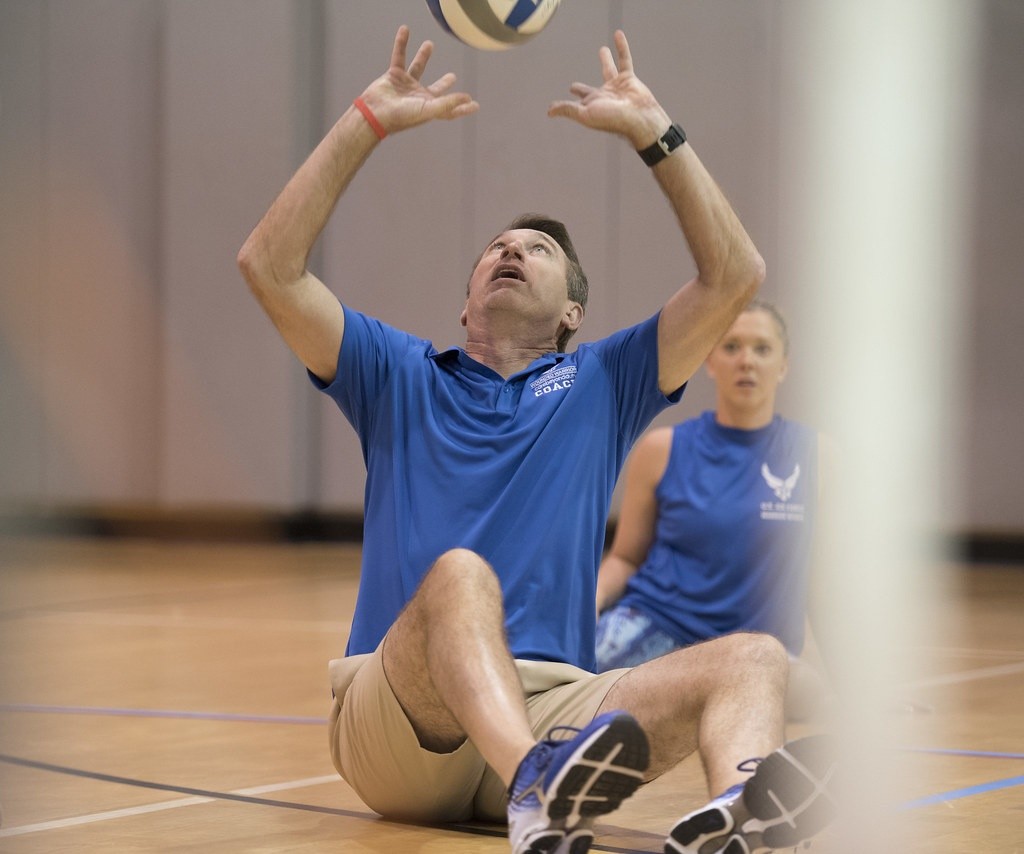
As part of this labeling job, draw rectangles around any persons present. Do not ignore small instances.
[596,301,833,722]
[236,22,844,854]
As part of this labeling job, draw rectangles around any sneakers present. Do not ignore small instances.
[506,712,651,854]
[663,734,865,854]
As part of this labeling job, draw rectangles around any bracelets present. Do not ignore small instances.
[636,119,687,168]
[346,95,389,140]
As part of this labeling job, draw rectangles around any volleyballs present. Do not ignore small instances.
[426,0,563,51]
